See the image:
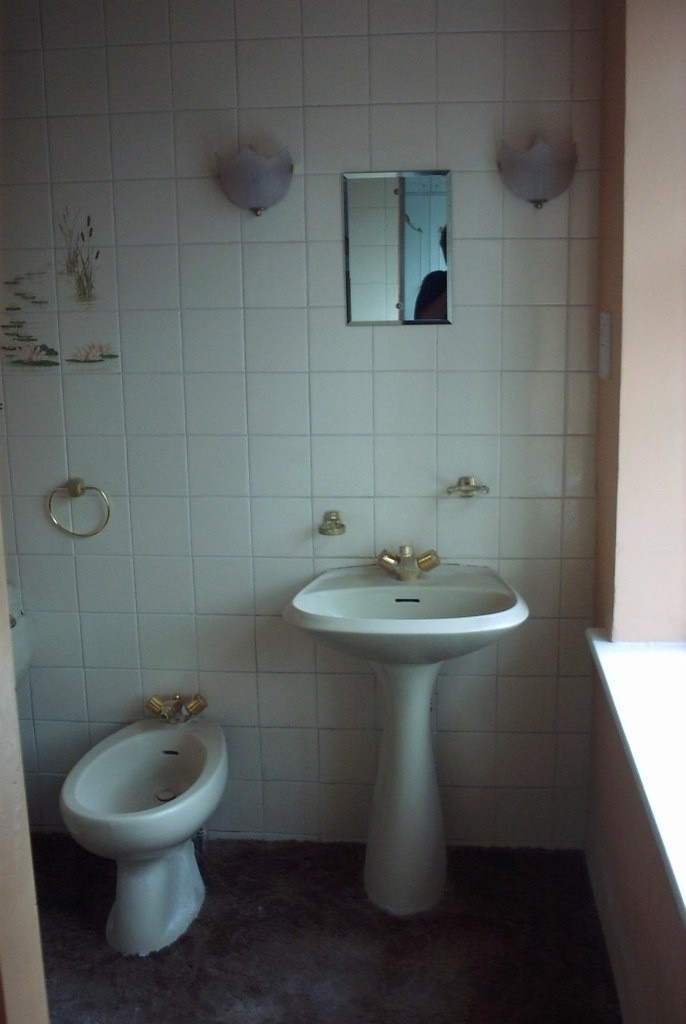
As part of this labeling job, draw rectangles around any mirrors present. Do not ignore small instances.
[342,169,453,326]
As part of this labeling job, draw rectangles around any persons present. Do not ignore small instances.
[414,224,447,319]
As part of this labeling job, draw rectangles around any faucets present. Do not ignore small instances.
[398,544,417,582]
[159,692,184,720]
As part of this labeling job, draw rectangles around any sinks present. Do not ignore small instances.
[282,565,529,666]
[61,718,230,863]
